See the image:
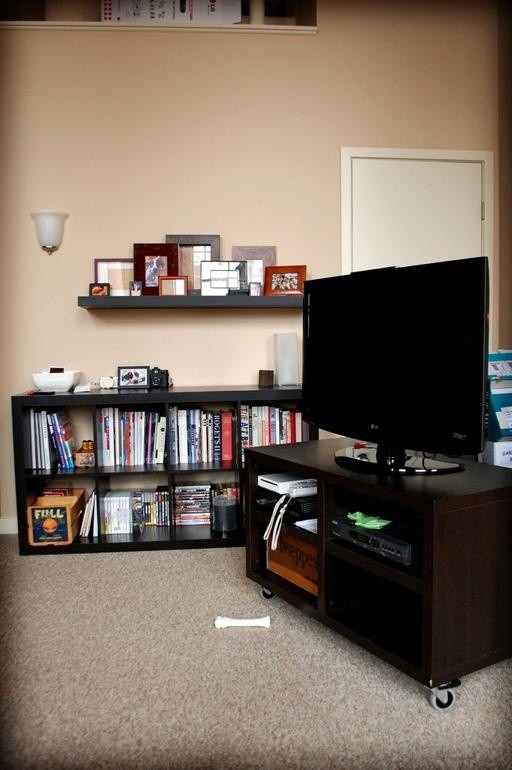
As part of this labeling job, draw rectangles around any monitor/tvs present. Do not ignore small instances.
[301,256,490,476]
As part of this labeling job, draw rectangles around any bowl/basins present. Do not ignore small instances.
[31,370,81,391]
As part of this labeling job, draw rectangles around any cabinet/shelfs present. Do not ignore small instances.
[244,439,511,714]
[9,384,320,555]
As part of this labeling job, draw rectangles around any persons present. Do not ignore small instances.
[272,273,298,290]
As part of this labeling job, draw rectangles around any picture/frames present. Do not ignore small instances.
[94,232,308,298]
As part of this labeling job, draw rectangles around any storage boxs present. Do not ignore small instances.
[482,348,512,468]
[25,487,87,549]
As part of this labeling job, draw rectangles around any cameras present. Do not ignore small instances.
[150,367,169,388]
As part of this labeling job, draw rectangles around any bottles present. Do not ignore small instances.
[259,369,273,387]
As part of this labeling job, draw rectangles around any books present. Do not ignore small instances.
[27,403,310,540]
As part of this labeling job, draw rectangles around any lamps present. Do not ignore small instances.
[30,207,70,255]
